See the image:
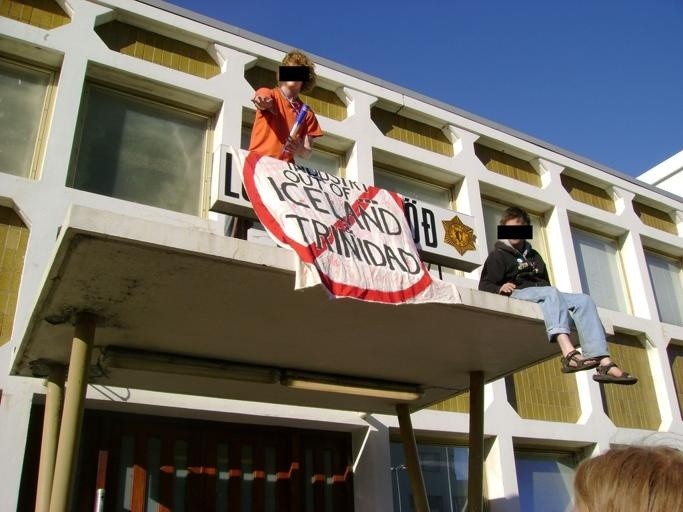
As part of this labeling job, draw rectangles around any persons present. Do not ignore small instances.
[247,51,323,163]
[478,206,638,385]
[568,443,683,512]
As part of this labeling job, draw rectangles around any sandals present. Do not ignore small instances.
[593,362,637,385]
[560,350,600,373]
